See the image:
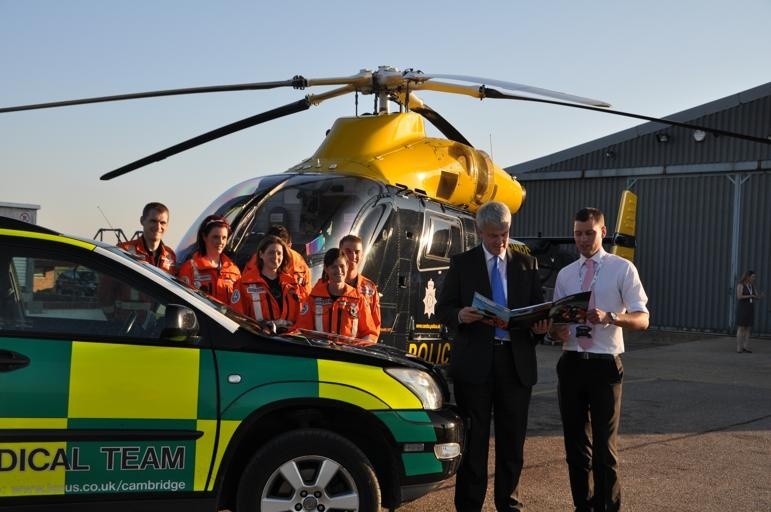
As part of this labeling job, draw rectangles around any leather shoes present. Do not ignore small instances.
[736,346,753,354]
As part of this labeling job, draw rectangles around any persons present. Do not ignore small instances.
[736,270,760,353]
[230,224,381,344]
[550,207,649,512]
[176,215,242,310]
[99,202,176,339]
[433,202,553,512]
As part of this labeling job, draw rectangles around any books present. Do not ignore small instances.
[471,290,591,332]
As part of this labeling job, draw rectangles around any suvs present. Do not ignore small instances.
[0,215,468,512]
[54,269,97,297]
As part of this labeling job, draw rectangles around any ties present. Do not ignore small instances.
[577,259,597,349]
[491,256,508,337]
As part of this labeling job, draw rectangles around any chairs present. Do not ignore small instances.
[267,205,291,238]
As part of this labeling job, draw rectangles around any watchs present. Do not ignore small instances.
[607,311,617,326]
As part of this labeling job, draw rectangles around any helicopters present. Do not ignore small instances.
[0,65,771,369]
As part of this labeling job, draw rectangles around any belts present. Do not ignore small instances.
[487,338,510,348]
[563,350,610,359]
